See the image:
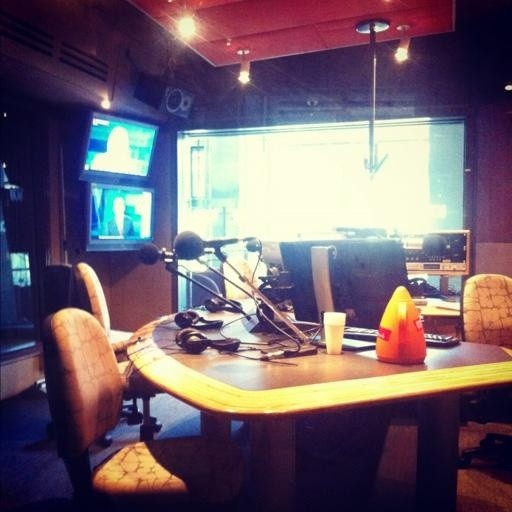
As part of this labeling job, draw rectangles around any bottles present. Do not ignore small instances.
[376,286,427,365]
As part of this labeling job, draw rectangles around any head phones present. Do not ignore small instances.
[408,278,427,295]
[204,296,242,313]
[174,310,223,329]
[176,328,241,353]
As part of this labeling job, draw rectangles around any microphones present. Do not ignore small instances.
[138,244,179,265]
[172,231,256,260]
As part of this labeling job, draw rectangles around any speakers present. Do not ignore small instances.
[133,72,197,119]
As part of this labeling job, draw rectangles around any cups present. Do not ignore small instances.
[323,312,347,355]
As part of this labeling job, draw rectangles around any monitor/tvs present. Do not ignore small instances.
[75,111,161,184]
[75,182,155,253]
[280,240,408,351]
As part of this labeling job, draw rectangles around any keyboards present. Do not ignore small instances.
[344,328,460,347]
